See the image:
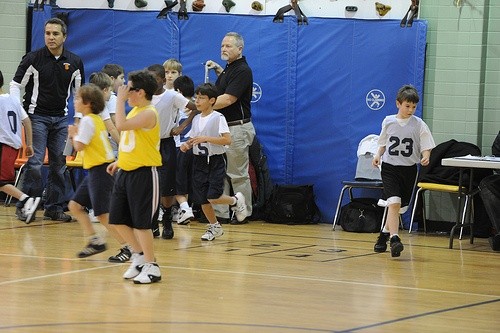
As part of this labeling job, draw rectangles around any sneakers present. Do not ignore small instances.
[374,235,389,253]
[231,192,247,221]
[16,207,34,222]
[44,207,72,222]
[23,197,41,224]
[391,242,403,257]
[78,207,224,283]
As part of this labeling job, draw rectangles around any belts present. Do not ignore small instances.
[228,118,250,126]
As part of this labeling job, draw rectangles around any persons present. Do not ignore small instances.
[67,84,134,263]
[0,69,41,224]
[106,68,161,284]
[172,75,195,225]
[372,84,436,257]
[205,31,256,224]
[89,72,119,222]
[181,82,248,241]
[479,130,500,251]
[10,18,85,222]
[147,64,200,241]
[101,64,125,126]
[158,59,182,221]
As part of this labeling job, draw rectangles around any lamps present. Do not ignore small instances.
[156,0,177,20]
[401,5,418,27]
[272,5,290,24]
[178,0,189,20]
[293,5,308,25]
[34,0,56,11]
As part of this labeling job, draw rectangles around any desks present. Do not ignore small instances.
[441,155,500,249]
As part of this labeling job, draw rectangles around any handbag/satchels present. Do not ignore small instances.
[339,198,385,233]
[264,185,319,224]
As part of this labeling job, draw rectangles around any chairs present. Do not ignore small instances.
[331,133,404,232]
[2,125,82,207]
[408,139,482,240]
